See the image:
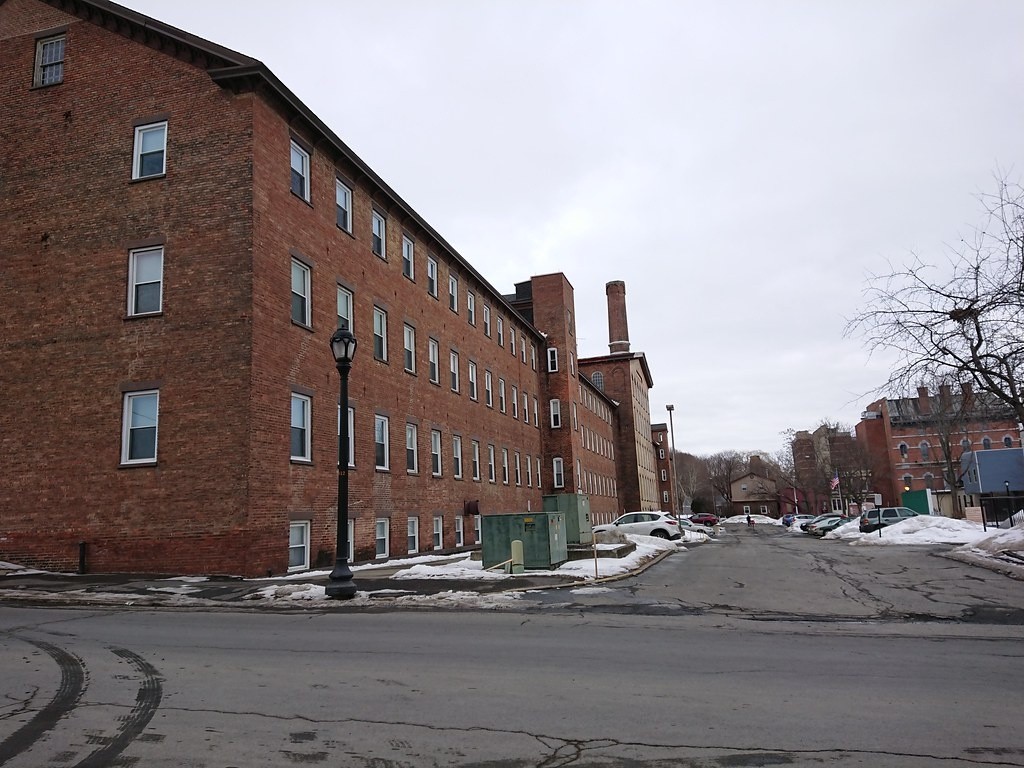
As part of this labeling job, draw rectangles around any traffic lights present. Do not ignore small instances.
[903,476,912,493]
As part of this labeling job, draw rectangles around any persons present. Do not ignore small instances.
[747,515,751,527]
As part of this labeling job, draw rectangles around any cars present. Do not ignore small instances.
[800,513,857,537]
[677,518,715,538]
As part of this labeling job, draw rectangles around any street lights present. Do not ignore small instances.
[666,404,681,526]
[325,322,359,598]
[710,476,716,515]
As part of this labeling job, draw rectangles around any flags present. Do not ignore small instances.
[831,470,839,490]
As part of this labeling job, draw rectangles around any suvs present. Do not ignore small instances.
[782,514,795,527]
[592,510,681,540]
[859,506,920,533]
[687,513,719,527]
[792,515,815,524]
